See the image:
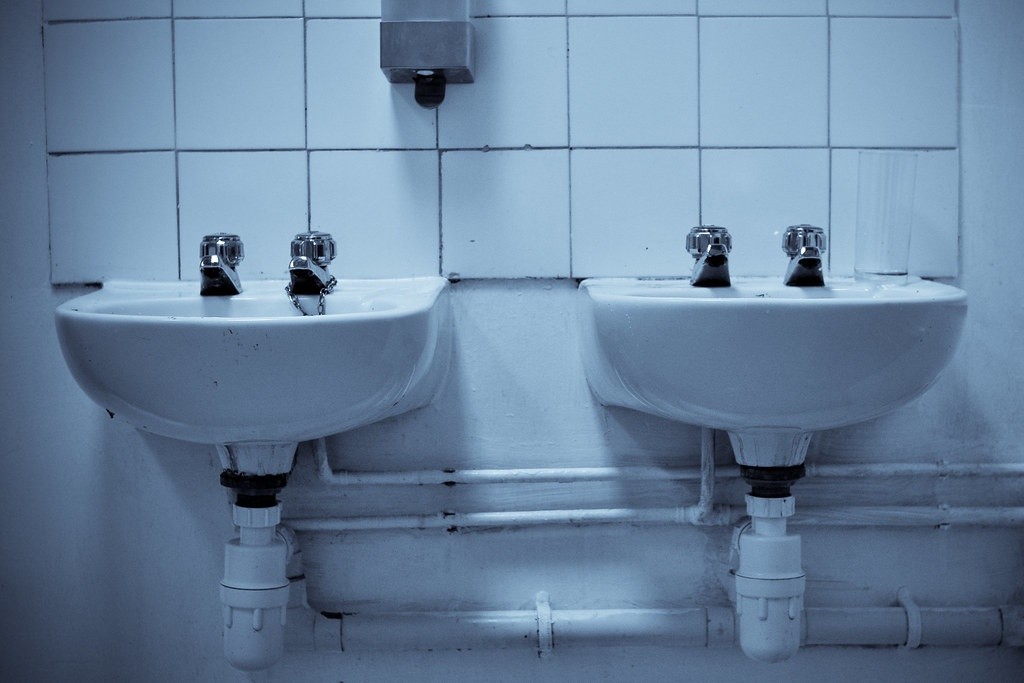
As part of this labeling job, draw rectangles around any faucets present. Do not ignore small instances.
[782,224,826,287]
[686,224,732,288]
[199,232,245,297]
[289,230,338,294]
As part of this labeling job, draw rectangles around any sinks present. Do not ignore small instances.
[576,287,970,433]
[54,294,451,445]
